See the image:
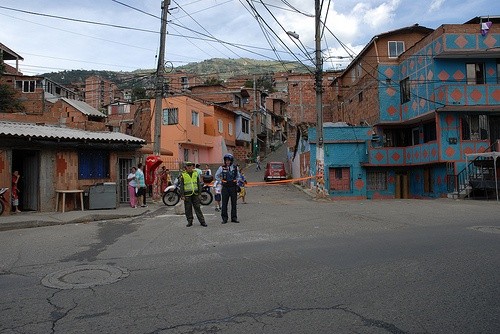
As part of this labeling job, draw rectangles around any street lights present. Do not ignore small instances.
[286,31,317,69]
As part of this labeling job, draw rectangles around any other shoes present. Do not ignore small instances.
[222,221,227,224]
[141,205,148,207]
[231,220,240,223]
[214,208,218,211]
[186,225,192,227]
[242,202,247,204]
[16,210,21,212]
[219,207,222,210]
[201,224,207,227]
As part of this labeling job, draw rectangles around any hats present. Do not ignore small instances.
[185,161,194,166]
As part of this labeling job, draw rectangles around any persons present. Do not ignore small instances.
[127,154,247,227]
[12,170,21,213]
[256,153,261,172]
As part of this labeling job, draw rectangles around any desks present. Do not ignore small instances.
[55,189,84,213]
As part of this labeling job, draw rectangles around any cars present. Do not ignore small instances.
[264,161,288,182]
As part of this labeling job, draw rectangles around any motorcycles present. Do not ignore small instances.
[163,175,213,206]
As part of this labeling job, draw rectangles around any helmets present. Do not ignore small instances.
[223,154,233,162]
